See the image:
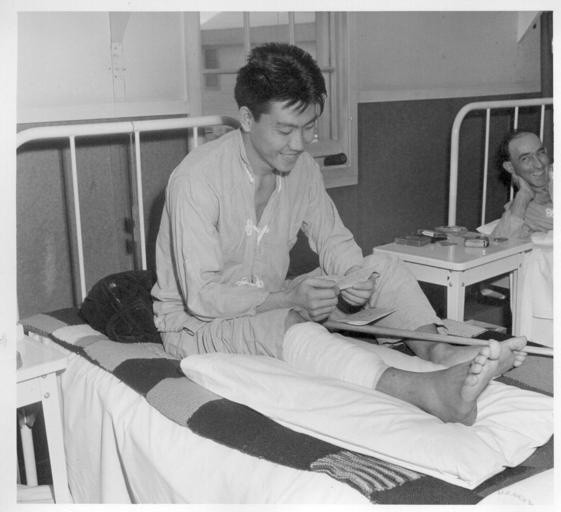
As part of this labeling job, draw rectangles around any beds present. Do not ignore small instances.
[449,97,554,350]
[16,116,554,505]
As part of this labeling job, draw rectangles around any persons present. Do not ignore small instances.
[491,127,553,241]
[151,43,528,427]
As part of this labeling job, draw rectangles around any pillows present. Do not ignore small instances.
[476,219,553,247]
[80,270,161,343]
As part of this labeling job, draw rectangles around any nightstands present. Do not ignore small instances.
[372,231,533,336]
[16,334,70,505]
